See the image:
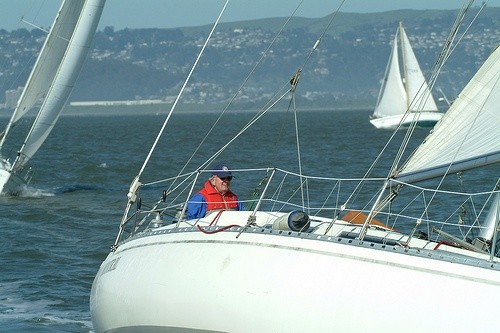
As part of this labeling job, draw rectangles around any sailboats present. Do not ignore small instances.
[89,1,500,333]
[369,20,444,129]
[1,0,105,198]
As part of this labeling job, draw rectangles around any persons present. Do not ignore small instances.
[186,164,245,220]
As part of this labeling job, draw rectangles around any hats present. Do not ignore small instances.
[211,164,236,179]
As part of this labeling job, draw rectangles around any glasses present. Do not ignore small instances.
[216,176,232,181]
